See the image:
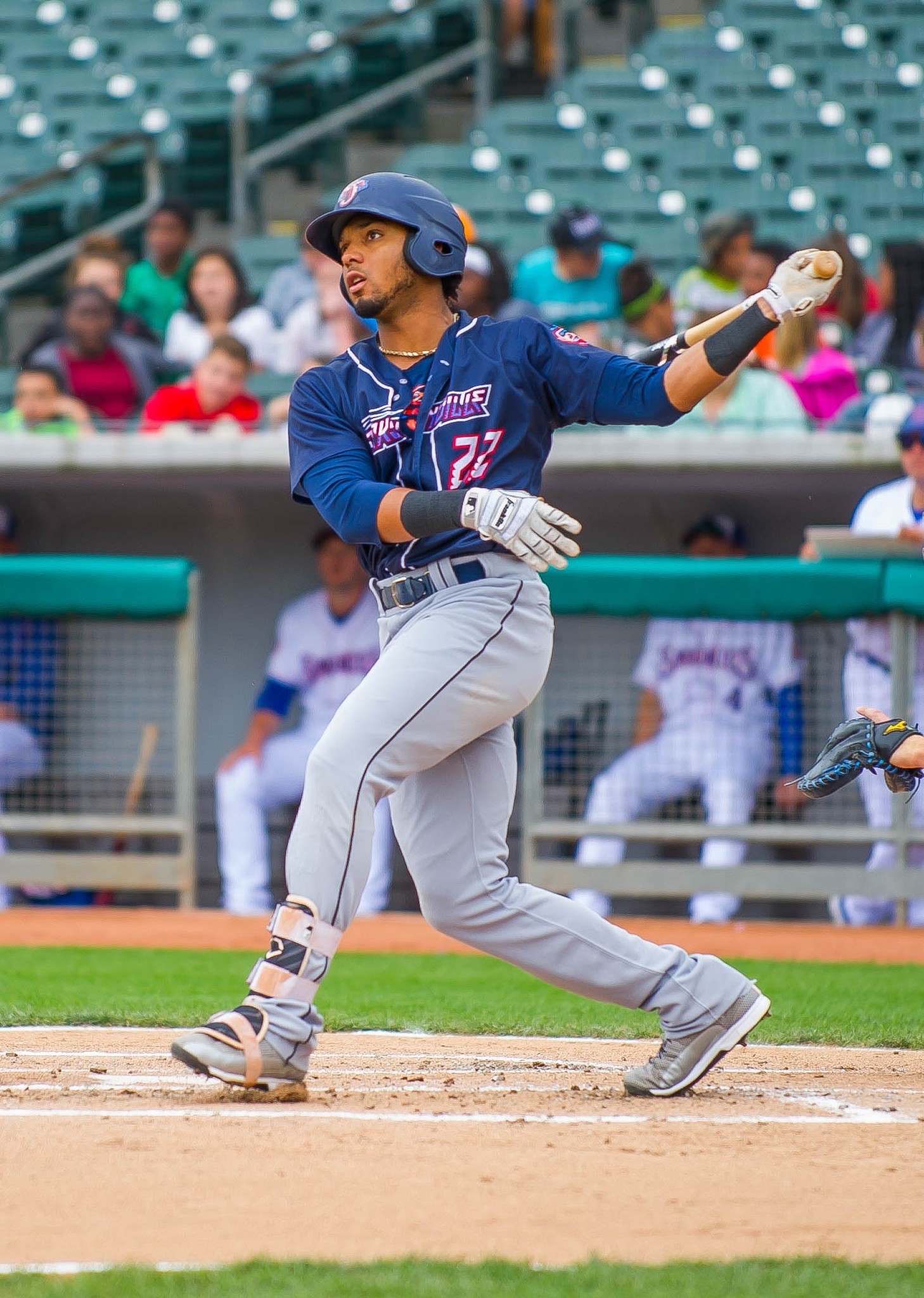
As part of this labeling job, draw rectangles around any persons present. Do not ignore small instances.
[827,404,924,929]
[213,525,396,920]
[0,196,924,440]
[172,169,844,1098]
[796,705,924,801]
[570,513,806,926]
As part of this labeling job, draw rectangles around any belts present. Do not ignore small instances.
[378,559,485,610]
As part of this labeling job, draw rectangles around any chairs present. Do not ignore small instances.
[0,0,924,432]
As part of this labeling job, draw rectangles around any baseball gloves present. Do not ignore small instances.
[796,716,924,800]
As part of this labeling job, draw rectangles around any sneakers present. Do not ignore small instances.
[171,1003,306,1092]
[622,983,771,1096]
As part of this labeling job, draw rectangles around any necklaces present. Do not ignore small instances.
[375,312,459,358]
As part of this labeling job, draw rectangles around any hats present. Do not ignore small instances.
[680,515,745,550]
[553,205,637,250]
[900,406,924,434]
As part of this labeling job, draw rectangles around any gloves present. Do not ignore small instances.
[763,248,843,324]
[460,487,580,571]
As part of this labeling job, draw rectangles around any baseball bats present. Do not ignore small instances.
[623,249,839,369]
[92,719,161,907]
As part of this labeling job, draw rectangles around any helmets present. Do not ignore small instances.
[305,172,467,305]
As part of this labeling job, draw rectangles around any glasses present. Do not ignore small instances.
[902,434,924,450]
